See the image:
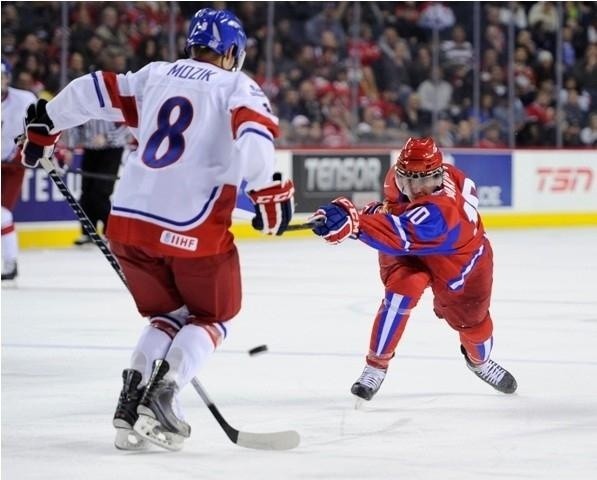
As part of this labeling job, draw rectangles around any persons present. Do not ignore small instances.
[15,6,296,438]
[1,60,39,283]
[307,136,518,401]
[63,62,130,245]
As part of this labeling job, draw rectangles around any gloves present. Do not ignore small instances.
[362,201,389,217]
[19,101,63,170]
[246,178,298,236]
[308,195,359,245]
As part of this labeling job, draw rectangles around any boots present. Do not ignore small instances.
[139,358,191,438]
[113,369,144,431]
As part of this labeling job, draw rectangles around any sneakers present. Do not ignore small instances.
[465,359,517,394]
[2,263,18,281]
[351,366,386,400]
[74,234,94,246]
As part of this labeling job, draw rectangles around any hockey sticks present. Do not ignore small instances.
[15,133,302,449]
[234,207,321,232]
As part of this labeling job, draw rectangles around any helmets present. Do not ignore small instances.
[0,60,11,76]
[393,135,443,197]
[185,7,247,71]
